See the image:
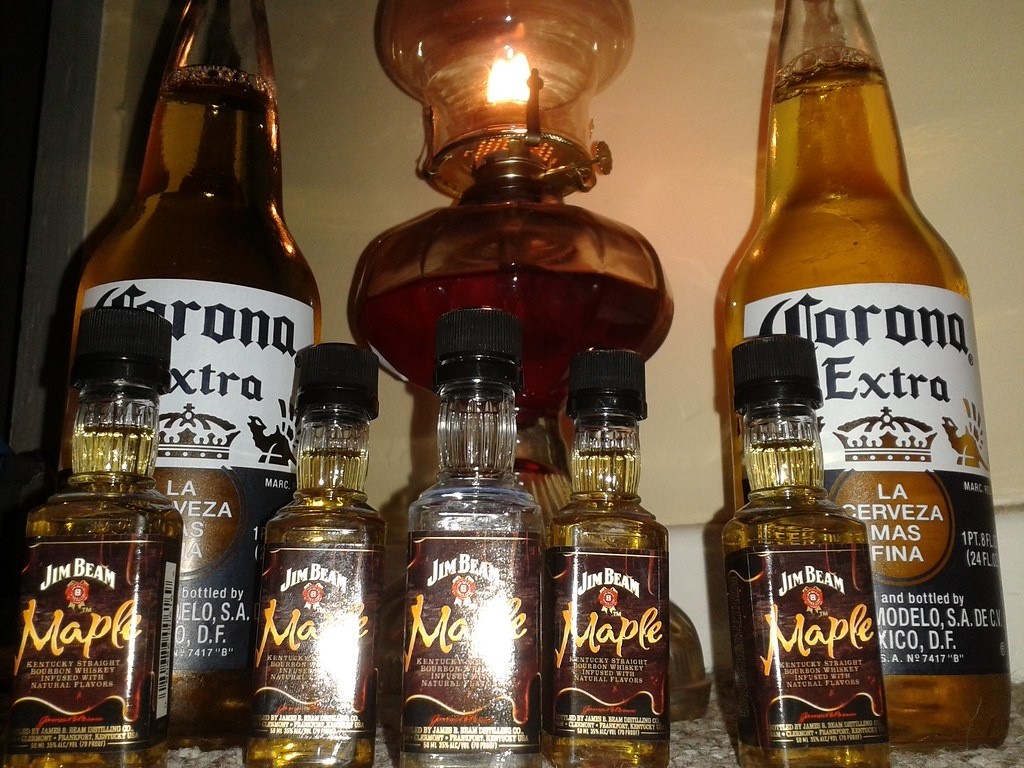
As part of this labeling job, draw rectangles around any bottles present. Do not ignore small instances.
[399,308,543,768]
[58,0,322,752]
[722,336,892,767]
[724,0,1011,752]
[245,343,388,767]
[541,347,670,767]
[2,306,184,768]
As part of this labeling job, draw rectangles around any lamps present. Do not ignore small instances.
[349,1,673,419]
